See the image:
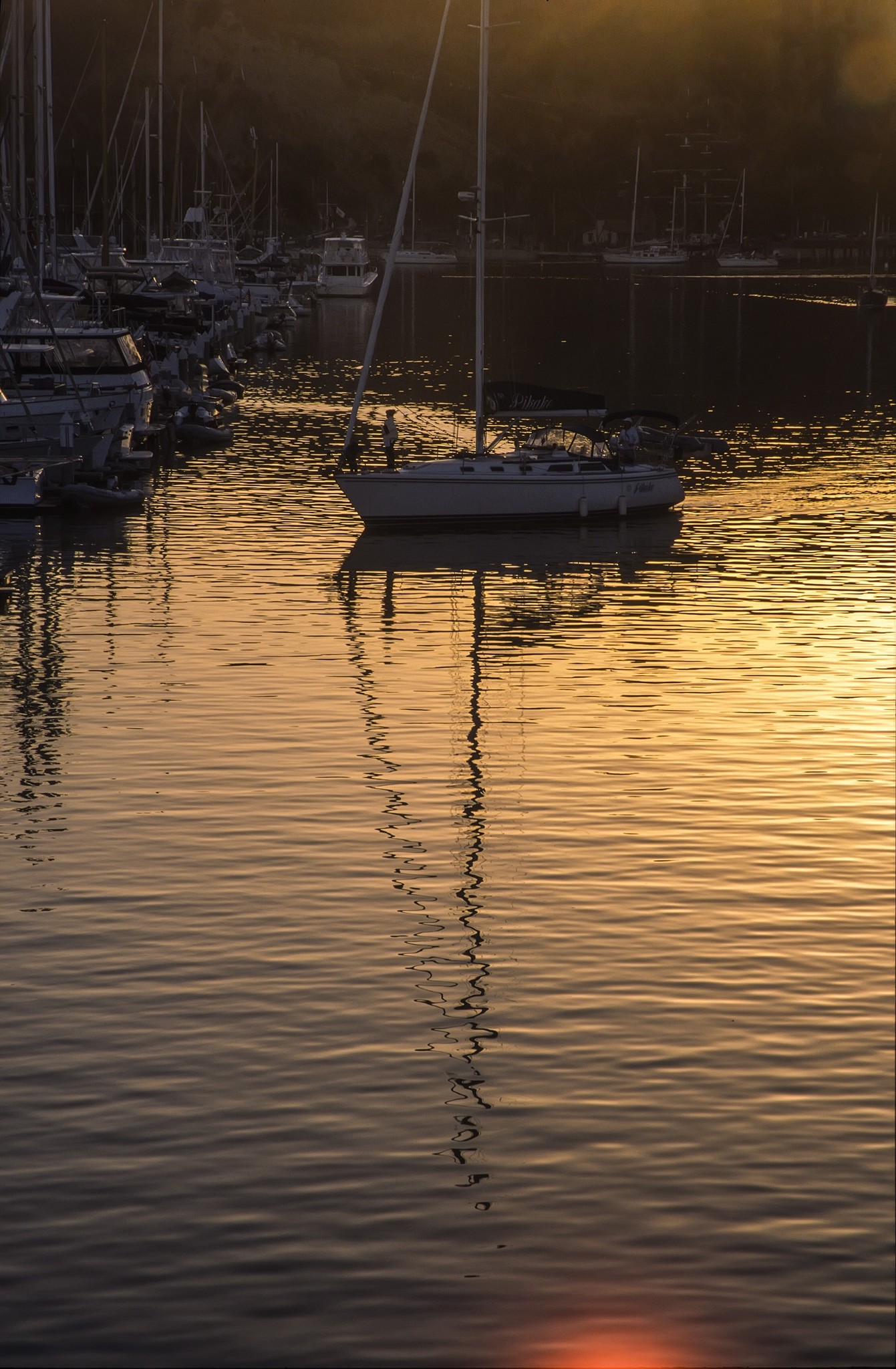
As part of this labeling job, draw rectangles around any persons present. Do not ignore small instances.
[618,418,641,466]
[379,409,399,473]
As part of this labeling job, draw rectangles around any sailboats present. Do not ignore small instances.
[384,163,460,265]
[599,145,721,266]
[1,0,385,524]
[854,189,889,309]
[317,1,687,535]
[713,168,780,268]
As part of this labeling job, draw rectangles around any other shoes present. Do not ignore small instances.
[384,468,394,472]
